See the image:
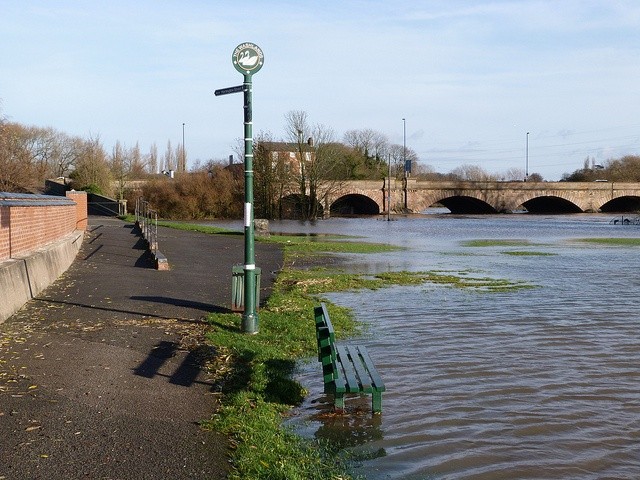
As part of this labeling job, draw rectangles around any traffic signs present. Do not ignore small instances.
[214,85,243,96]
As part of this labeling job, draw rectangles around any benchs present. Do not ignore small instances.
[314,302,385,416]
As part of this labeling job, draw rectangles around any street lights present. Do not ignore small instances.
[526,132,530,176]
[402,119,406,178]
[183,122,186,171]
